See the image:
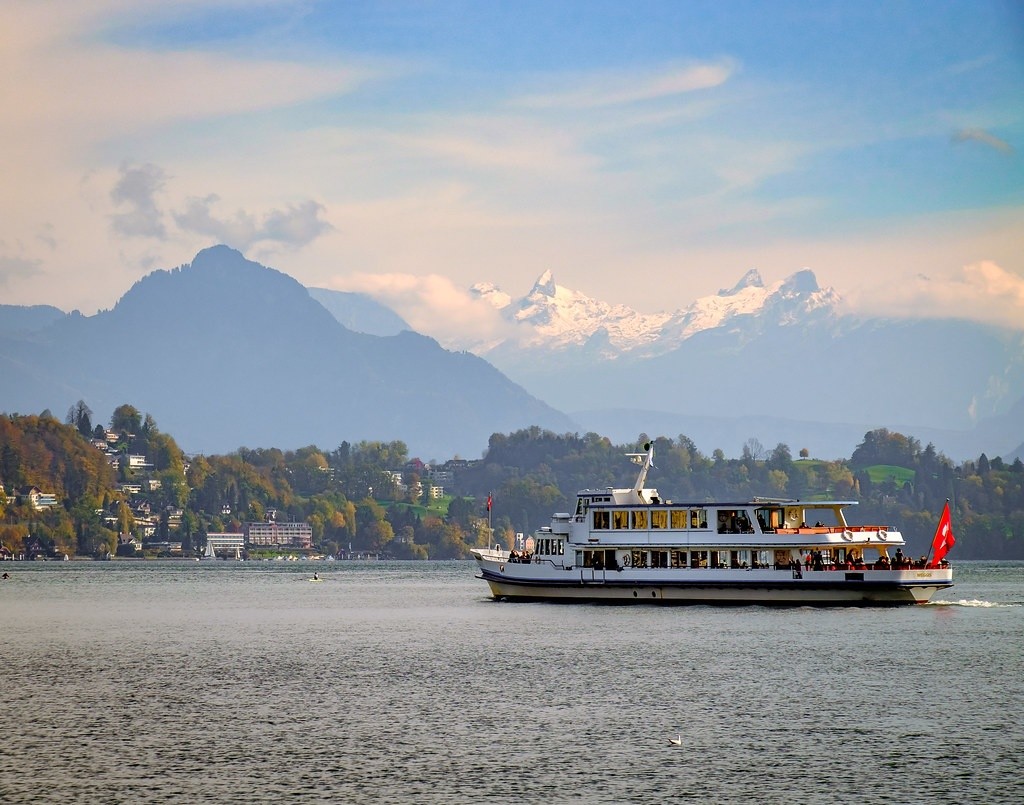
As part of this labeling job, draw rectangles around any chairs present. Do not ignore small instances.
[806,562,922,571]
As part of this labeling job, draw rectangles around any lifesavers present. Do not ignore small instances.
[850,548,859,561]
[775,550,786,562]
[788,508,799,521]
[877,529,888,541]
[843,529,853,541]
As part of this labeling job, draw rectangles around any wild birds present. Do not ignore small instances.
[313,572,318,580]
[1,573,10,579]
[668,735,681,746]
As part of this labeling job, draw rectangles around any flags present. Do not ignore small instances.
[485,496,492,511]
[930,504,955,569]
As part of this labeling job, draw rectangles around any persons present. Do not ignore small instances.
[313,572,318,580]
[0,572,9,579]
[506,550,531,563]
[734,514,929,569]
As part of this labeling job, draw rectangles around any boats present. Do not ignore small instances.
[471,439,956,607]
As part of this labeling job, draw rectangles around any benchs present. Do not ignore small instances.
[775,526,835,534]
